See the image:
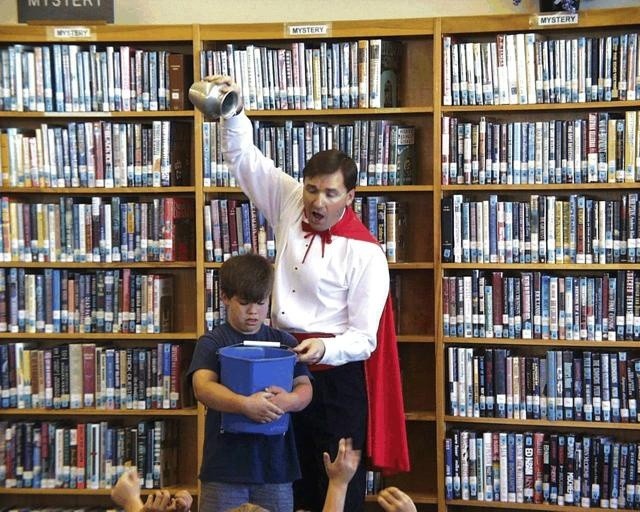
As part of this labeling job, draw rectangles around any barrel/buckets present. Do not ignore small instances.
[214,341,297,439]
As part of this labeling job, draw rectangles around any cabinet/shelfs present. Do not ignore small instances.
[0,6,640,512]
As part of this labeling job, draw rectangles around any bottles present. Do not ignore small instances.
[188,80,241,120]
